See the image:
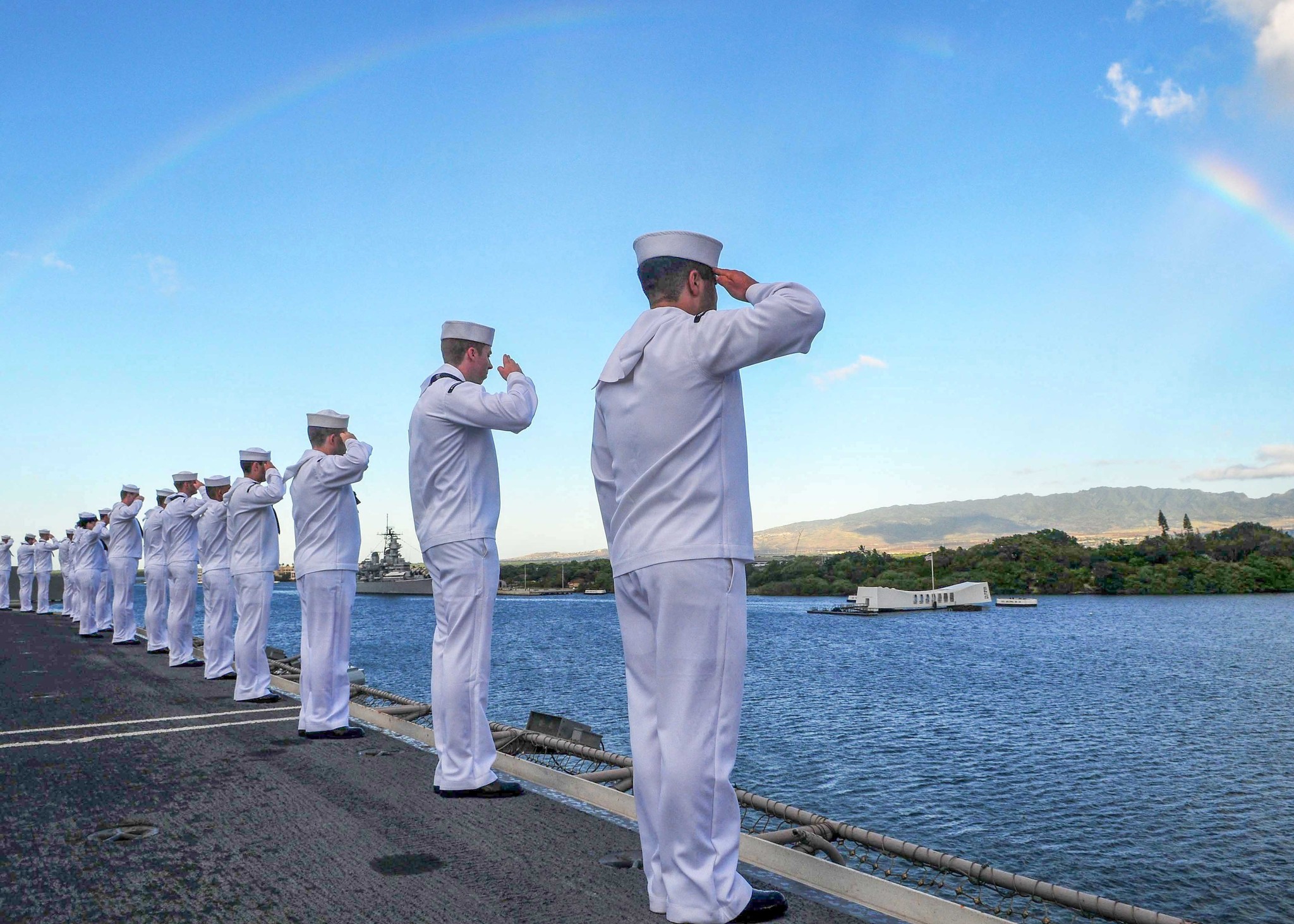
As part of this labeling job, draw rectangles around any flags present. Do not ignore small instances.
[924,554,932,561]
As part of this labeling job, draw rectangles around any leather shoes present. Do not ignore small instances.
[434,779,524,798]
[147,646,170,654]
[98,628,114,632]
[234,687,281,703]
[297,725,364,739]
[0,607,53,615]
[113,639,141,645]
[62,614,80,623]
[209,671,237,680]
[170,659,205,667]
[80,633,104,638]
[726,887,789,923]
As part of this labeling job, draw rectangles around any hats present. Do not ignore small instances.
[306,409,349,429]
[38,529,50,534]
[172,471,198,481]
[1,535,11,539]
[633,231,723,269]
[239,447,271,461]
[78,511,96,518]
[156,488,175,497]
[441,320,495,346]
[75,520,81,523]
[65,529,74,534]
[122,484,140,493]
[26,533,36,539]
[204,475,230,487]
[98,508,112,514]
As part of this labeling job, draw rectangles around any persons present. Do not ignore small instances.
[588,229,827,924]
[142,488,178,655]
[191,474,238,681]
[94,507,114,632]
[222,446,287,702]
[57,529,75,617]
[16,534,39,612]
[0,535,14,611]
[34,528,59,615]
[162,470,211,667]
[281,410,374,740]
[409,321,537,799]
[69,511,109,638]
[107,483,144,644]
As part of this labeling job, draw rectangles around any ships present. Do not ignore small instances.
[355,512,433,595]
